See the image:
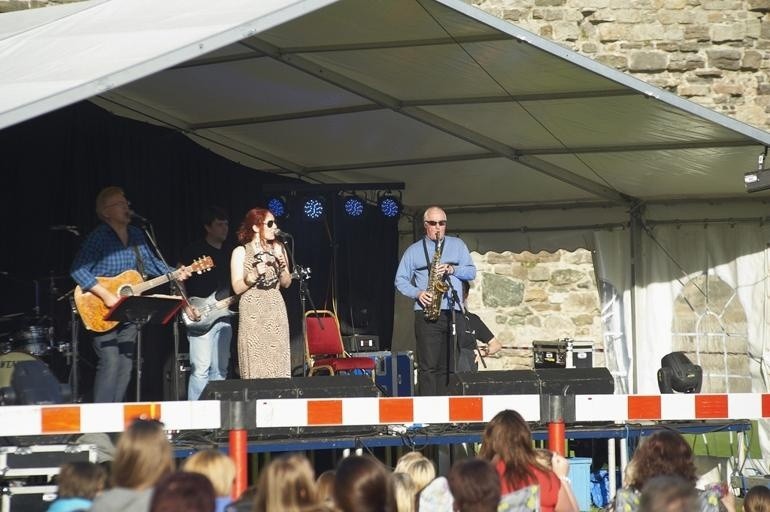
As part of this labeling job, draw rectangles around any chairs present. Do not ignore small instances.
[303,310,376,387]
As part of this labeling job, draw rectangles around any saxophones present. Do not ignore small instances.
[423,232,450,322]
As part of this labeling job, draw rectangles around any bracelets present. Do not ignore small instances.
[558,475,570,481]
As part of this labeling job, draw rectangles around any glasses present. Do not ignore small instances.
[425,220,446,226]
[263,220,276,228]
[102,200,131,208]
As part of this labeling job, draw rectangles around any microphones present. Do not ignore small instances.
[274,229,292,240]
[128,209,147,224]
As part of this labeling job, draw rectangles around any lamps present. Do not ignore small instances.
[657,351,703,395]
[341,190,368,221]
[301,194,328,224]
[266,196,287,218]
[744,147,770,192]
[377,190,403,218]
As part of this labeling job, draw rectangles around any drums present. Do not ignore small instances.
[14,325,54,356]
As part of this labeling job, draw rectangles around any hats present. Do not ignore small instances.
[73,432,115,464]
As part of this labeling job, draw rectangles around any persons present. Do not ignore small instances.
[92,418,171,511]
[173,207,231,400]
[449,458,502,512]
[151,471,216,512]
[48,461,107,512]
[254,459,330,512]
[460,280,502,372]
[615,428,720,512]
[391,473,418,512]
[395,206,476,396]
[332,455,398,512]
[393,451,436,493]
[478,409,580,512]
[224,452,315,512]
[231,208,291,378]
[720,485,770,512]
[180,449,236,512]
[313,470,344,512]
[70,187,192,403]
[639,476,702,511]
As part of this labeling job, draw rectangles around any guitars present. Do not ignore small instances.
[182,291,239,336]
[74,255,214,333]
